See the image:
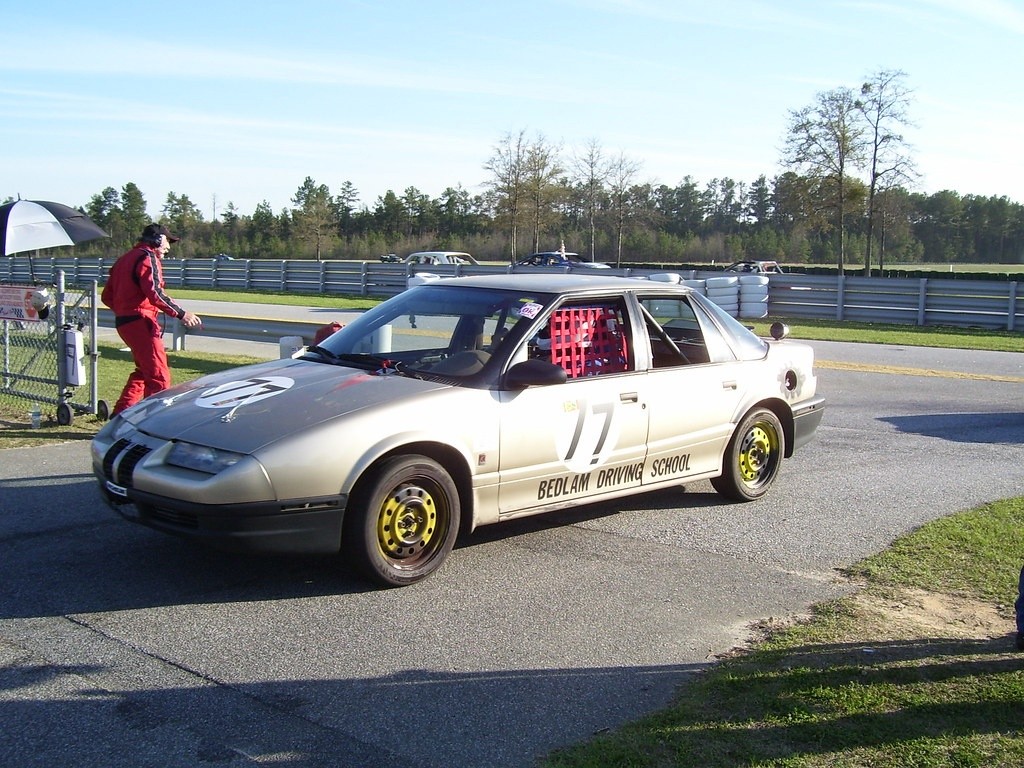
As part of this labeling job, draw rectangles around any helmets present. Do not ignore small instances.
[536,305,595,351]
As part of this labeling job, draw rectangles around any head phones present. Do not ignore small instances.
[137,234,162,248]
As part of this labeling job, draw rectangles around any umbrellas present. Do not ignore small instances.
[0,196,110,285]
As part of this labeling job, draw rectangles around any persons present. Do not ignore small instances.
[536,307,624,378]
[101,223,202,420]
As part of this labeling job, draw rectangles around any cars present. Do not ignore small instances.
[380,253,402,264]
[404,249,480,266]
[90,271,828,589]
[510,250,611,270]
[722,259,806,275]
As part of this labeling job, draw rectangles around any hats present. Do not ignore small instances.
[142,223,179,244]
[31,286,51,320]
